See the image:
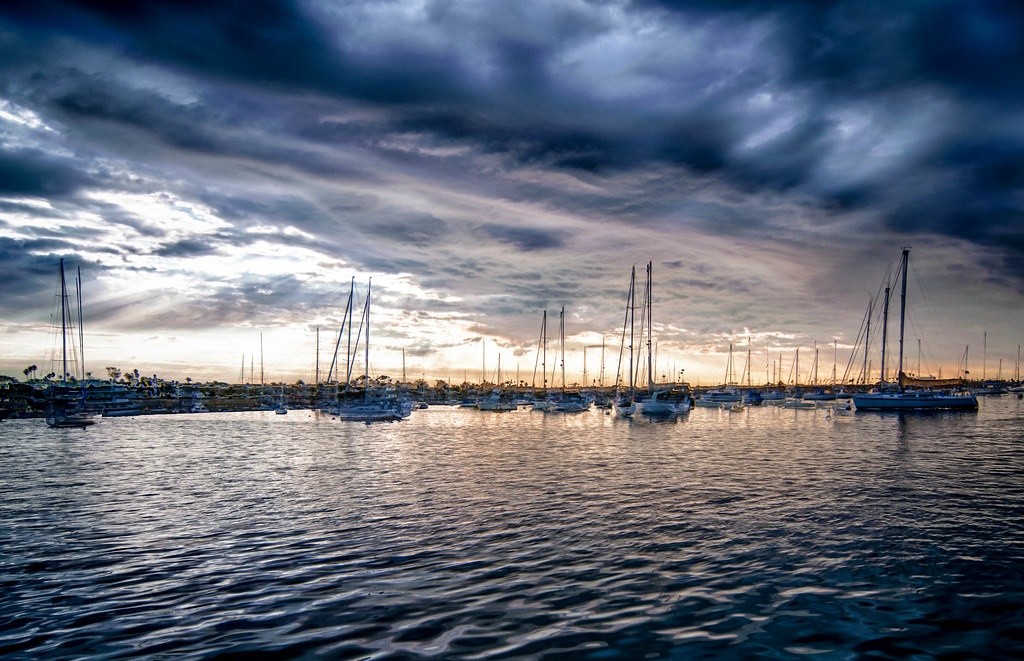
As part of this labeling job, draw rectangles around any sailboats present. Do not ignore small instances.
[0,247,1024,424]
